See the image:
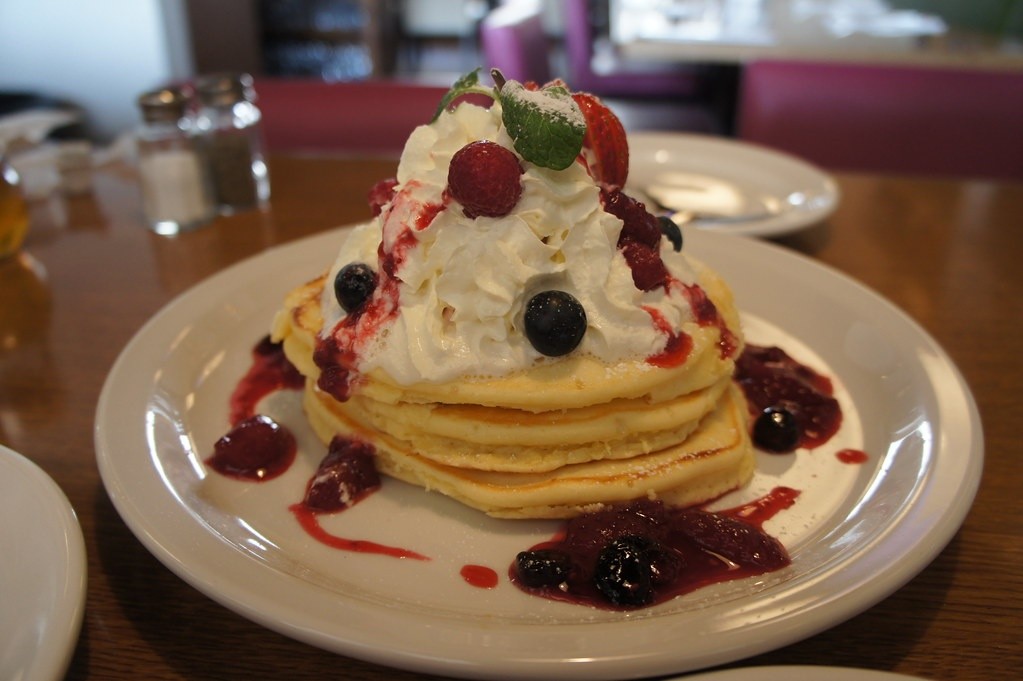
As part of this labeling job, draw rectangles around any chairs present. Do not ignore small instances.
[738,61,1018,184]
[564,0,701,97]
[186,78,497,152]
[482,0,551,89]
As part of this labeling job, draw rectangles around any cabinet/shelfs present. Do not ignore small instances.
[186,0,568,84]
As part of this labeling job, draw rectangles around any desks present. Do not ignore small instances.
[609,0,1023,70]
[1,152,1023,681]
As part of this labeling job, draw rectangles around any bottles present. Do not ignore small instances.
[190,72,274,206]
[138,78,194,127]
[133,123,219,234]
[0,155,53,357]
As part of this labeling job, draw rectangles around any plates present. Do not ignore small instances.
[0,444,89,681]
[92,217,989,681]
[619,130,840,239]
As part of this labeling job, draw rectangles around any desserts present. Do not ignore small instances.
[272,66,755,522]
